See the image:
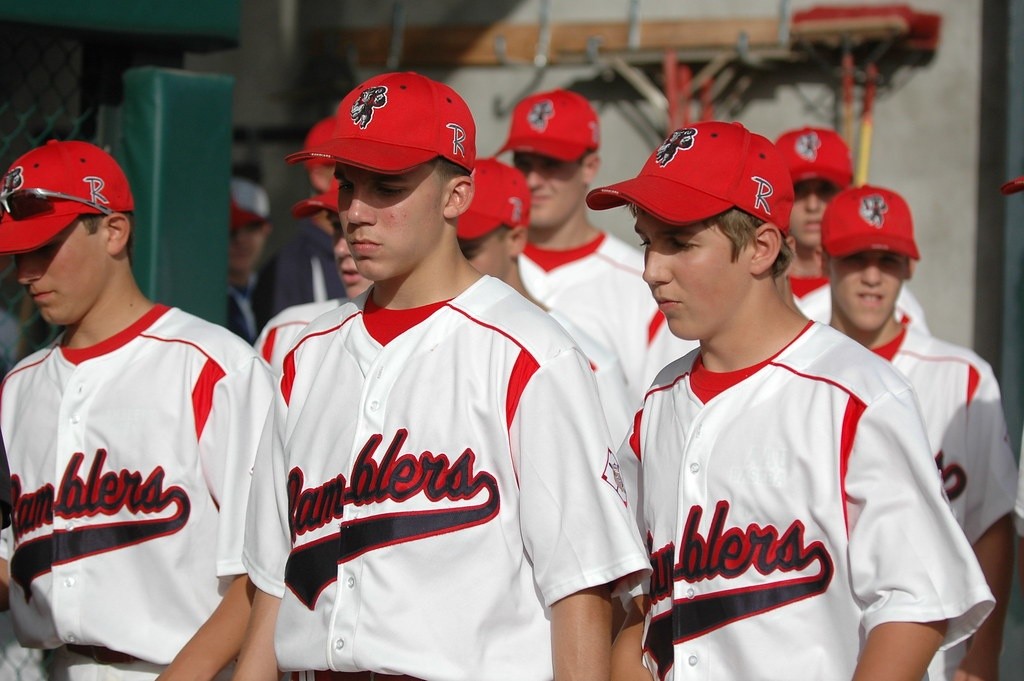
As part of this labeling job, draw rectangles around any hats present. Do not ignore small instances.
[584,119,794,238]
[292,178,339,221]
[283,71,477,175]
[776,126,853,192]
[302,116,337,167]
[821,184,920,261]
[494,88,600,163]
[0,139,134,256]
[231,179,271,225]
[458,157,532,240]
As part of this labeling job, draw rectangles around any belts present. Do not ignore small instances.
[64,643,136,665]
[290,669,425,680]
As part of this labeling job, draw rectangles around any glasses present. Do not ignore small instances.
[0,187,113,218]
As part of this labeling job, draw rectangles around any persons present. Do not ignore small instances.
[774,125,1023,680]
[229,117,373,374]
[585,121,996,680]
[231,71,653,681]
[0,138,276,680]
[457,87,700,641]
